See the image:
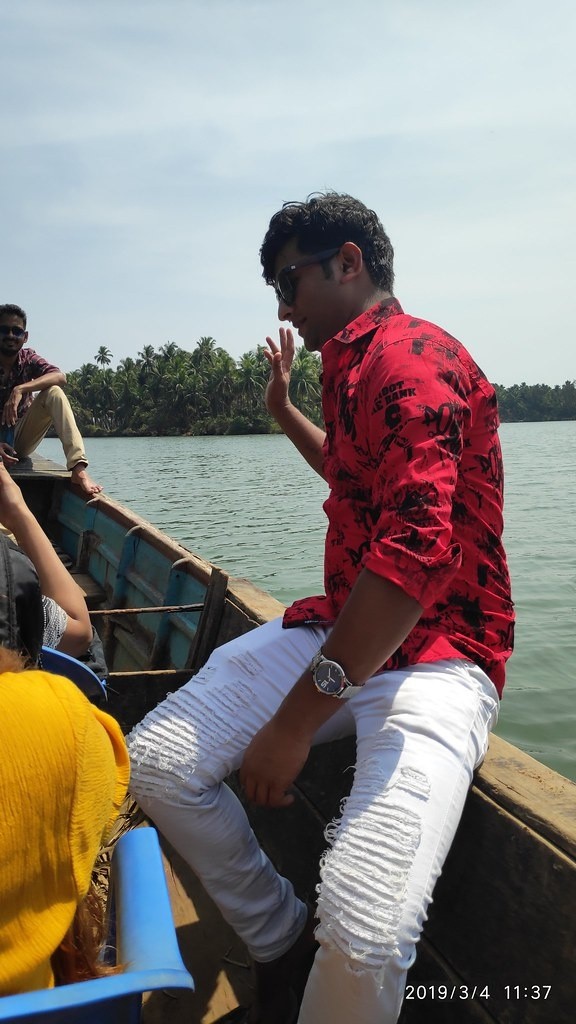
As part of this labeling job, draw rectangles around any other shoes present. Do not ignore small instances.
[251,899,321,1024]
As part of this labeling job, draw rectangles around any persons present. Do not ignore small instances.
[125,191,516,1024]
[0,303,104,494]
[0,455,132,1024]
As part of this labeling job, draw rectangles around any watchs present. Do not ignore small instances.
[310,646,366,699]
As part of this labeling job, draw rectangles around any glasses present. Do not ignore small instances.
[274,245,341,304]
[0,324,24,335]
